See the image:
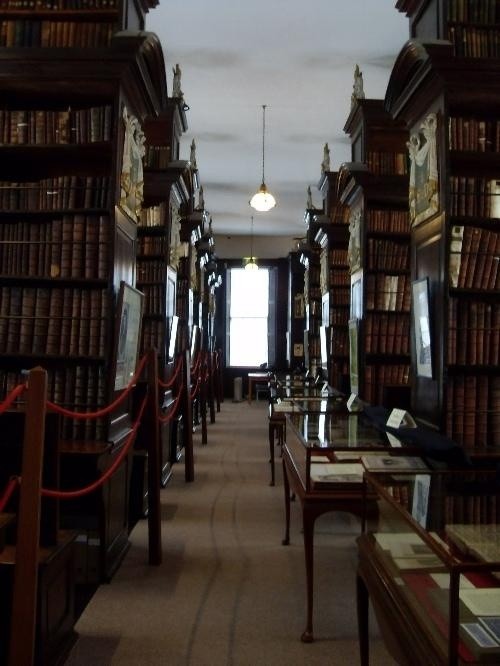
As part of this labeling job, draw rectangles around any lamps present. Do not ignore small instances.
[244,218,259,274]
[249,105,276,211]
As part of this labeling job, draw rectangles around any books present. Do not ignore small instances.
[1,1,199,444]
[293,1,499,535]
